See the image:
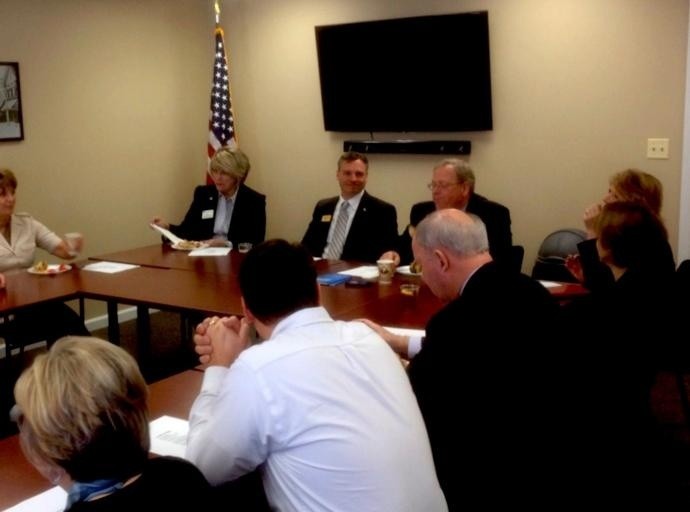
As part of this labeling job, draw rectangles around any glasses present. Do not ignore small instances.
[410,261,423,272]
[429,181,452,190]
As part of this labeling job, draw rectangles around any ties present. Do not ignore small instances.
[325,202,350,260]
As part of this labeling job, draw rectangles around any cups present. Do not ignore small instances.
[377,259,395,284]
[64,233,82,257]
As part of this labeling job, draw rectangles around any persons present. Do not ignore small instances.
[379,159,513,274]
[300,151,398,262]
[186,238,450,511]
[564,169,664,292]
[148,147,266,248]
[10,334,223,512]
[347,208,561,512]
[562,201,675,481]
[0,167,92,345]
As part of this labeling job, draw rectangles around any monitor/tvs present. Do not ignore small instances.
[314,9,494,134]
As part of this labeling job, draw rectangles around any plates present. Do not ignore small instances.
[171,243,209,250]
[396,265,421,277]
[28,263,72,276]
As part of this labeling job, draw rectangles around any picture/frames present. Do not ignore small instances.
[0,60,26,142]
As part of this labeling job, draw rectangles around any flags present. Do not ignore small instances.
[206,1,238,189]
